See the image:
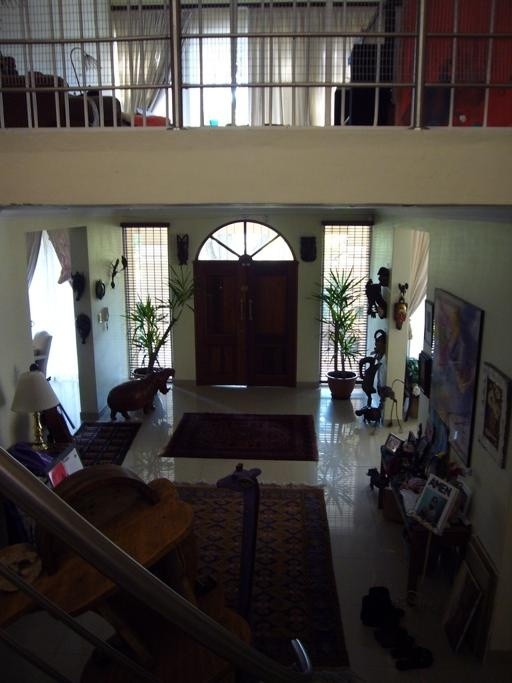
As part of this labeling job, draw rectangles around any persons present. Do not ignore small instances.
[418,496,438,518]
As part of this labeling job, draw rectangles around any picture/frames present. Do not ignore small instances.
[478,362,510,468]
[428,287,485,468]
[424,299,434,347]
[384,432,405,455]
[418,351,431,398]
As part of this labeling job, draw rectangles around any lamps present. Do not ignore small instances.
[11,371,60,450]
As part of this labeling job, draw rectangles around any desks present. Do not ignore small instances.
[378,445,470,608]
[0,441,77,551]
[1,463,253,682]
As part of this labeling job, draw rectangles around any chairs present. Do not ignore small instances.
[0,52,121,126]
[32,332,51,379]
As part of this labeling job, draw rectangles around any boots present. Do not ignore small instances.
[360,585,404,624]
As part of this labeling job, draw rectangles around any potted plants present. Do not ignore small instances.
[120,261,196,378]
[305,269,367,398]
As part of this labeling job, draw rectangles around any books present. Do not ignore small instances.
[412,472,458,533]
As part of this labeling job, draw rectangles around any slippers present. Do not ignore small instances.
[372,625,433,671]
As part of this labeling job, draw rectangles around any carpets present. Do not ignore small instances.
[158,412,319,461]
[171,482,349,670]
[73,422,141,465]
